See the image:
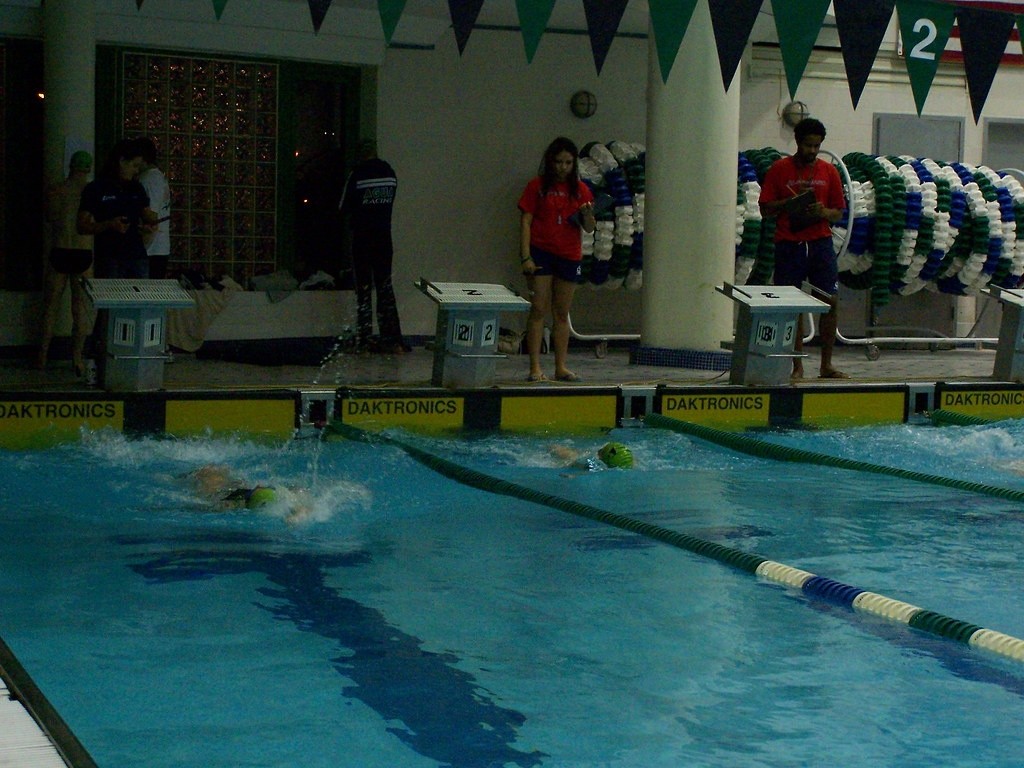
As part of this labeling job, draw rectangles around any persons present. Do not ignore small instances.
[517,137,597,382]
[339,139,405,354]
[759,118,851,378]
[35,140,171,377]
[188,468,280,510]
[551,443,633,469]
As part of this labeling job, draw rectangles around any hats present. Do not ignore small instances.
[70,151,93,173]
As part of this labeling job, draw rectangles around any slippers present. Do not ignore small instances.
[817,370,851,378]
[528,372,547,382]
[555,371,582,383]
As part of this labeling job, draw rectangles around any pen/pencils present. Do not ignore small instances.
[533,265,544,270]
[786,183,799,196]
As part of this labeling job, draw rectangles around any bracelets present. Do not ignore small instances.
[521,255,531,264]
